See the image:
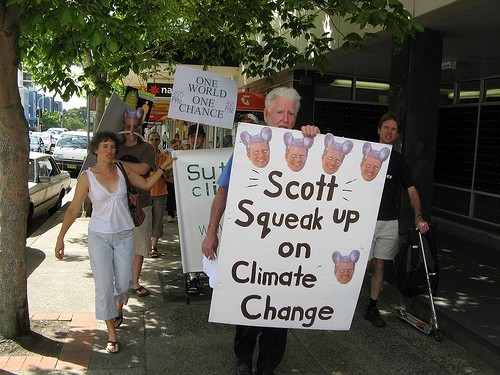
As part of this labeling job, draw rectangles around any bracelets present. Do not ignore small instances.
[158,167,165,172]
[415,215,422,218]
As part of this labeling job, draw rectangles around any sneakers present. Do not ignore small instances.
[364,308,385,327]
[235,359,252,375]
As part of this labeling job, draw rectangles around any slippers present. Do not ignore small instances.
[114,307,123,328]
[134,286,148,297]
[106,341,120,353]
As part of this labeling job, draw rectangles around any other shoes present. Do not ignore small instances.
[167,216,175,222]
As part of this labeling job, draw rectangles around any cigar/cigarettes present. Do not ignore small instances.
[207,256,211,261]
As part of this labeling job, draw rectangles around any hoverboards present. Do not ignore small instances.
[395,226,442,342]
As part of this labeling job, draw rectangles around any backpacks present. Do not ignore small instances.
[394,230,438,297]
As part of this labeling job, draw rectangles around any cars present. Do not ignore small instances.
[29,127,94,179]
[27,151,73,233]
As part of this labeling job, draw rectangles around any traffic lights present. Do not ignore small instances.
[35,109,39,117]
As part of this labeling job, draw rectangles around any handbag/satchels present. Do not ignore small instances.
[116,161,145,227]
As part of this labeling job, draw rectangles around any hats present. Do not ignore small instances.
[188,124,205,138]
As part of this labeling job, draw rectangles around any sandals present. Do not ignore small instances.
[151,249,158,258]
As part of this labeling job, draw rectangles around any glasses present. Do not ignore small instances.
[150,137,160,141]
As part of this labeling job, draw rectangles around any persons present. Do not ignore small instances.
[113,108,156,297]
[362,111,430,327]
[145,131,172,258]
[201,86,320,375]
[162,132,168,150]
[163,123,206,222]
[55,132,178,354]
